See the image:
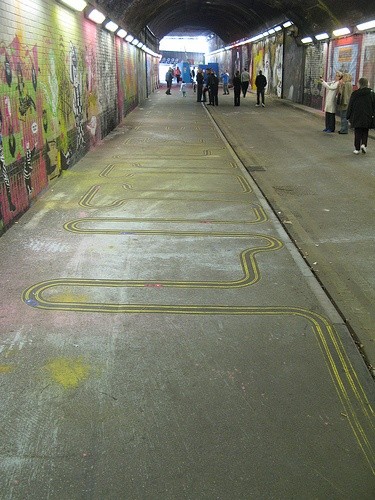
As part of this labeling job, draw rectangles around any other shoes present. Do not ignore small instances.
[360,144,367,153]
[261,103,265,107]
[322,129,334,134]
[338,130,348,134]
[352,149,360,155]
[256,104,259,107]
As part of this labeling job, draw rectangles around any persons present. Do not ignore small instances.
[190,68,242,107]
[345,78,375,154]
[335,73,352,134]
[255,70,267,108]
[319,71,344,133]
[179,81,187,97]
[165,66,182,95]
[241,68,250,98]
